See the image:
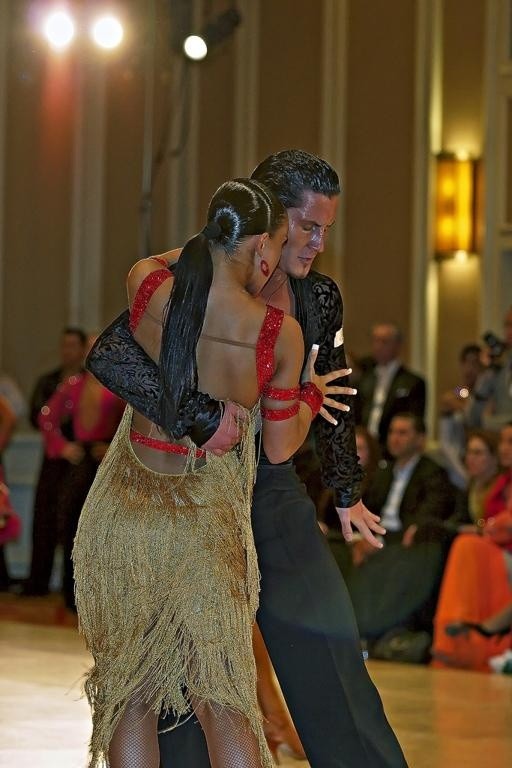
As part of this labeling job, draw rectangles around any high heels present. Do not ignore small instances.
[262,716,306,764]
[467,621,512,644]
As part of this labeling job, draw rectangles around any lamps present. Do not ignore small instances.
[431,148,478,262]
[183,6,243,61]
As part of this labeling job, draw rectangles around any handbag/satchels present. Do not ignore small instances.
[372,628,431,663]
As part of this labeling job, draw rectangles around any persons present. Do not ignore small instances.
[307,324,512,675]
[0,326,129,608]
[83,148,407,768]
[66,175,356,768]
